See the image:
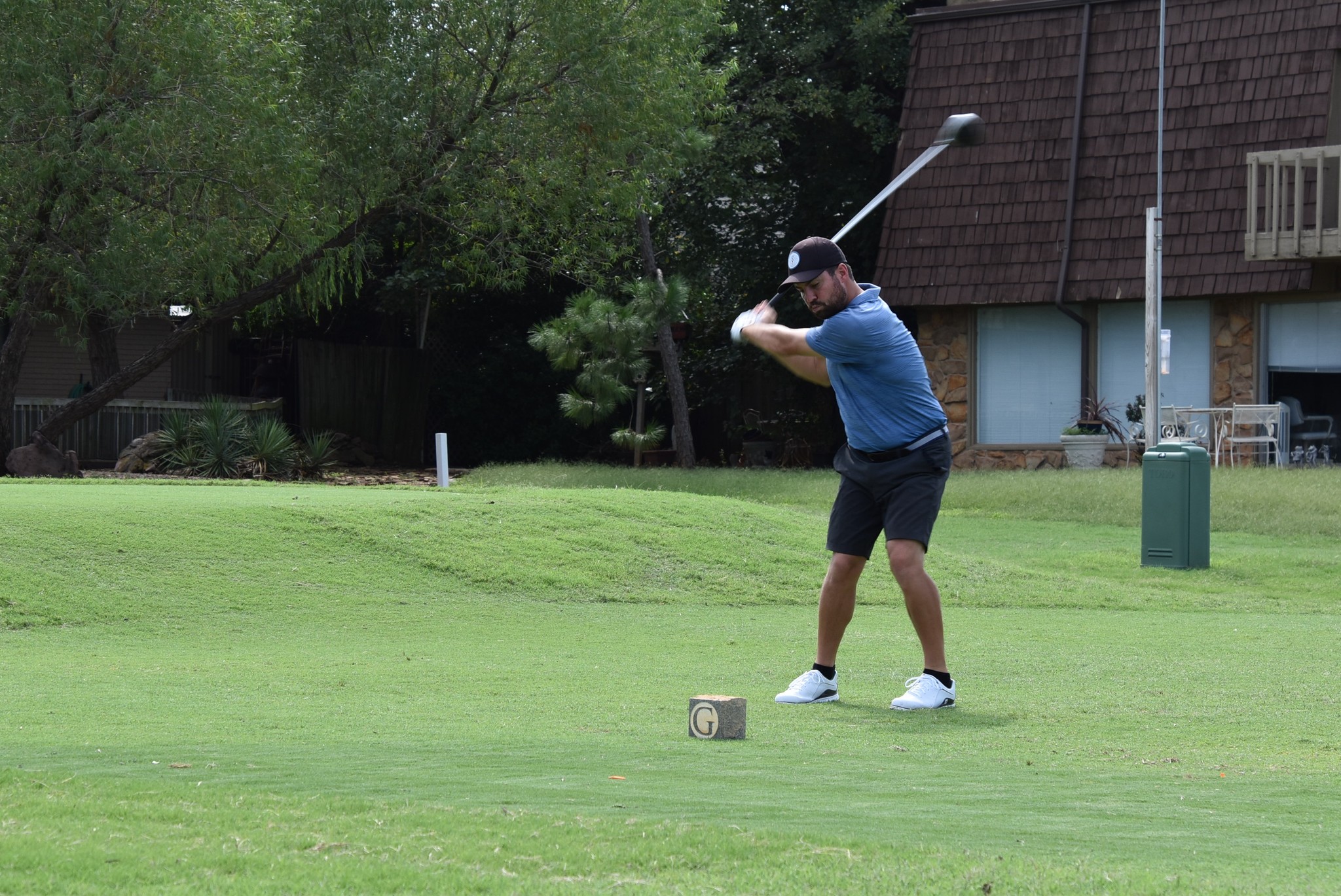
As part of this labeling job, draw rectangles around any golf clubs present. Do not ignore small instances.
[765,111,983,308]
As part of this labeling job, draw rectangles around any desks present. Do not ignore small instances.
[1177,408,1233,469]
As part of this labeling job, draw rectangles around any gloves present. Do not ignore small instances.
[730,310,756,342]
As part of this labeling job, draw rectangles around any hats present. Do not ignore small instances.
[777,237,847,294]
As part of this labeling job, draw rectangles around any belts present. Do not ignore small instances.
[853,448,911,463]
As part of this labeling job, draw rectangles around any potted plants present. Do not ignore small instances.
[1059,428,1109,470]
[1069,396,1131,448]
[1125,393,1186,467]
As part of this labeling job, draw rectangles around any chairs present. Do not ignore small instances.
[1175,404,1193,443]
[1124,405,1210,467]
[1223,401,1285,472]
[1277,395,1338,463]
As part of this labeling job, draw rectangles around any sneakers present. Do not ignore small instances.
[889,673,955,712]
[775,668,841,706]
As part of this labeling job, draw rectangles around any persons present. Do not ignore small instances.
[732,237,956,712]
[1269,395,1305,463]
